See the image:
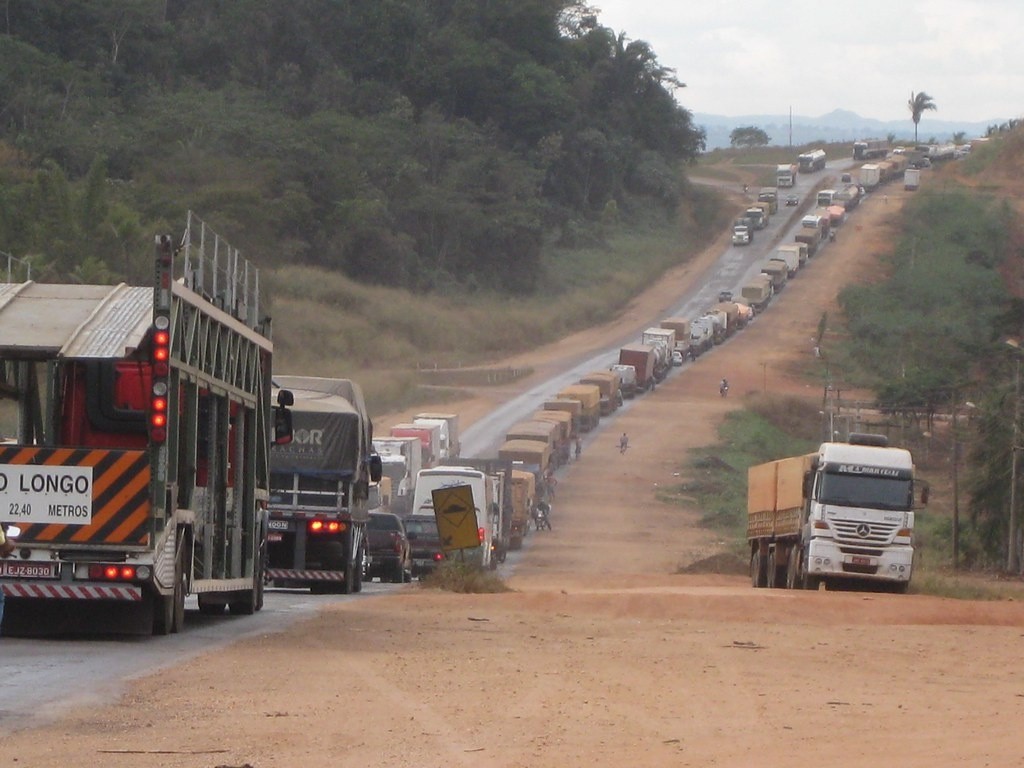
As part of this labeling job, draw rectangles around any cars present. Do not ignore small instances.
[785,194,800,207]
[893,146,905,154]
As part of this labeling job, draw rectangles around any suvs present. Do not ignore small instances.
[362,513,445,584]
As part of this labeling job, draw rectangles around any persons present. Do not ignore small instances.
[620,433,628,454]
[617,388,623,406]
[719,379,729,393]
[651,377,656,391]
[575,437,582,460]
[534,499,553,532]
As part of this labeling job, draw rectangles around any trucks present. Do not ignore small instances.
[903,169,920,192]
[853,140,889,161]
[544,318,692,433]
[794,165,880,252]
[743,241,829,313]
[878,154,907,181]
[734,187,780,245]
[914,144,972,163]
[746,432,932,594]
[797,148,826,174]
[264,375,383,594]
[502,410,577,552]
[683,291,755,358]
[367,414,506,569]
[0,209,295,639]
[775,163,796,186]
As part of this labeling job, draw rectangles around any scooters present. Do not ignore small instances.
[621,444,628,455]
[720,386,729,397]
